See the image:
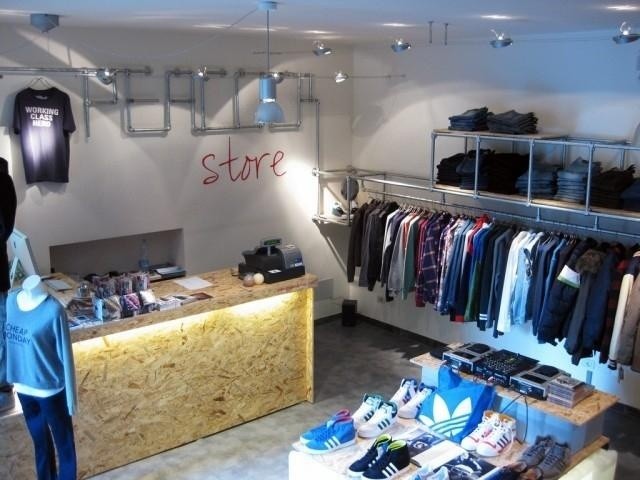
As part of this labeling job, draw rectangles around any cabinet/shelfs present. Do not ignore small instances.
[313,127,640,247]
[288,342,618,480]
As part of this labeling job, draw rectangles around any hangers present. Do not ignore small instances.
[24,66,58,91]
[363,188,640,263]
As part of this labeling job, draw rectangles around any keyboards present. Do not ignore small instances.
[45,280,73,292]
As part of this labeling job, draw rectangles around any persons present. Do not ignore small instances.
[4,274,78,480]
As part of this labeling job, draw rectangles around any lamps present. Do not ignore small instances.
[612,21,640,45]
[391,38,410,54]
[96,67,122,85]
[193,66,211,83]
[253,0,285,122]
[489,29,513,49]
[274,71,286,82]
[311,40,331,57]
[333,67,348,83]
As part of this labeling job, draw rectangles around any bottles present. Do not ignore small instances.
[138,238,150,271]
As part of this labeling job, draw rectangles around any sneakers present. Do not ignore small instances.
[348,434,409,480]
[522,434,569,478]
[409,466,450,480]
[301,393,398,454]
[486,459,544,480]
[389,378,437,418]
[461,409,516,457]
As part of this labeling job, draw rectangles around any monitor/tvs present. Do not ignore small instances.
[8,228,42,288]
